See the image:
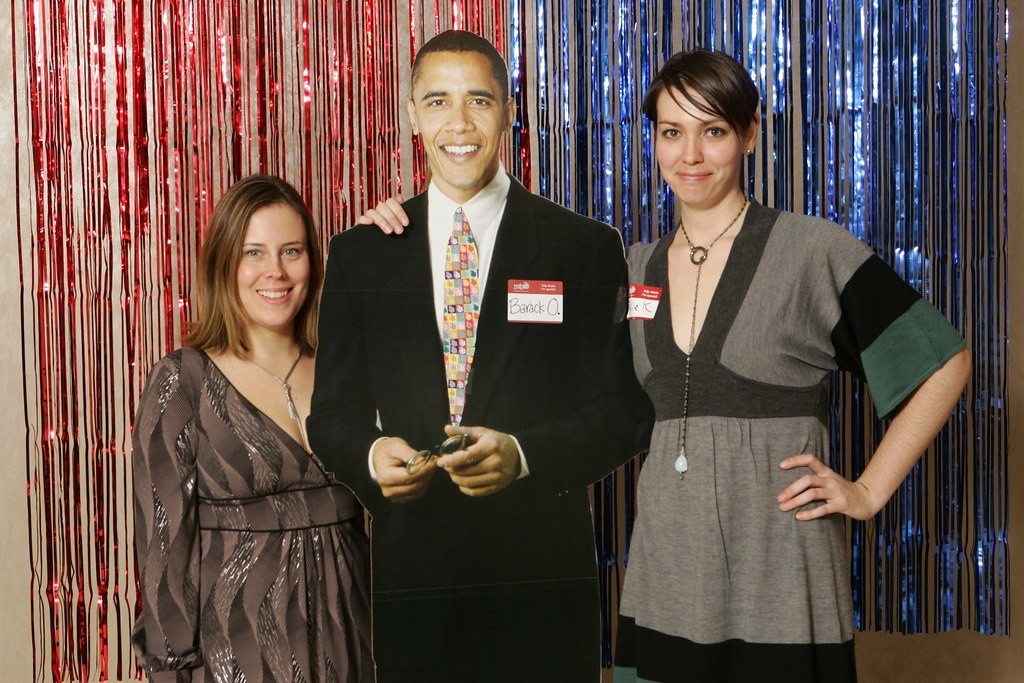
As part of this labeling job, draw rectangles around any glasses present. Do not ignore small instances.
[405,433,473,474]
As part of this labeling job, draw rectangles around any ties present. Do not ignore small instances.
[442,207,480,428]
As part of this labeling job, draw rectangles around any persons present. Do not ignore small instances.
[358,49,972,683]
[307,29,654,682]
[129,175,359,683]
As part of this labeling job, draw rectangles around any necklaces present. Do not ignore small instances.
[674,196,748,480]
[237,349,301,420]
